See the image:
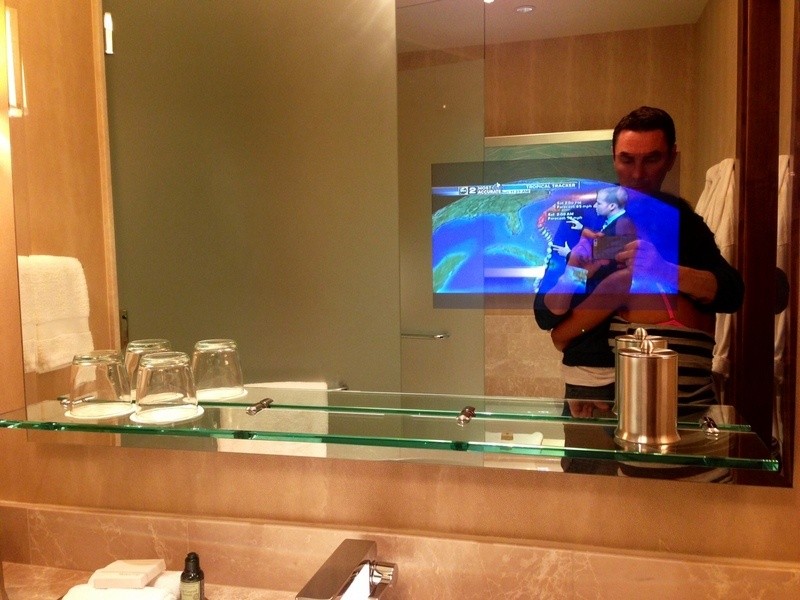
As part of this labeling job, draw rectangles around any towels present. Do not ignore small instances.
[17,252,99,376]
[59,583,178,600]
[215,376,330,462]
[88,564,188,598]
[690,153,790,385]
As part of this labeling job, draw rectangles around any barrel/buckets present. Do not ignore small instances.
[612,328,667,415]
[615,339,681,444]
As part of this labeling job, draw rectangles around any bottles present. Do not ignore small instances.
[178,552,205,600]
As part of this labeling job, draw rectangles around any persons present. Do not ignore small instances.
[534,105,745,477]
[546,184,638,293]
[550,195,737,491]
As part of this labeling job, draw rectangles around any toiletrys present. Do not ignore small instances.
[177,550,208,600]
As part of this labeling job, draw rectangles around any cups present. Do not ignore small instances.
[136,351,199,422]
[192,339,244,401]
[124,338,170,400]
[69,350,132,418]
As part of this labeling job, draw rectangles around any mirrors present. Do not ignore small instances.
[6,0,799,490]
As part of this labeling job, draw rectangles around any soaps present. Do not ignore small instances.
[94,556,167,590]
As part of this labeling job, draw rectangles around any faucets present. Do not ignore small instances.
[293,537,398,600]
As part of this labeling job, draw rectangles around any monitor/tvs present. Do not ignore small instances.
[429,153,682,309]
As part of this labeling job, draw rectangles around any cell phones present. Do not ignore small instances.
[590,234,640,261]
